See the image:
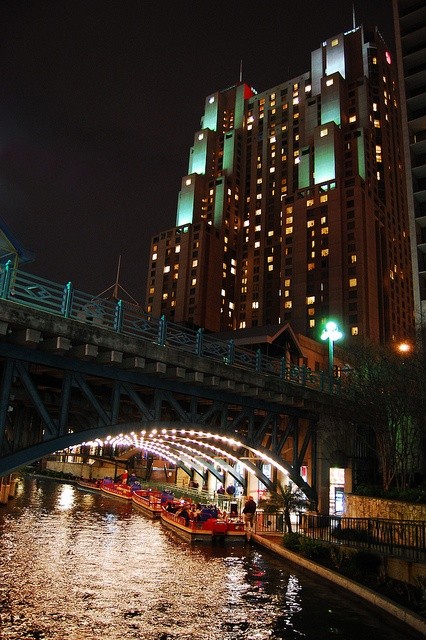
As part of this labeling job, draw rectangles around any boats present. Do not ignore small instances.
[100,479,141,499]
[160,502,245,535]
[77,479,101,490]
[132,490,184,511]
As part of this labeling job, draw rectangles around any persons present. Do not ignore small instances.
[166,501,218,522]
[244,495,255,532]
[218,485,224,494]
[193,480,198,488]
[126,472,138,485]
[188,479,193,487]
[226,482,236,499]
[121,470,127,485]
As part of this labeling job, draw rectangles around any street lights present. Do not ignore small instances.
[320,321,342,395]
